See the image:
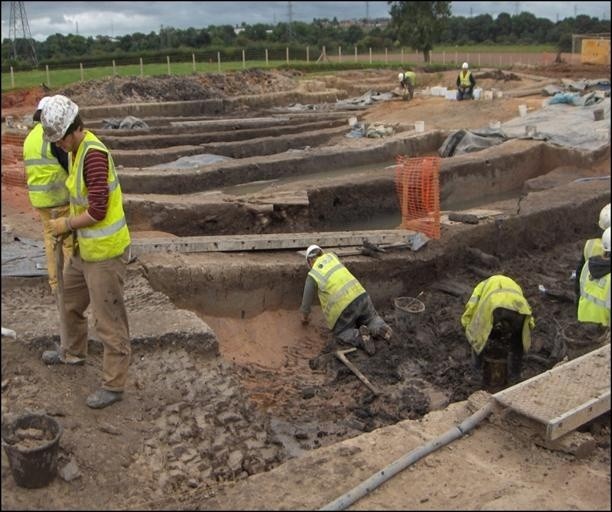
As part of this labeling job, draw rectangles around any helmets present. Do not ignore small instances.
[37,96,52,110]
[306,245,324,269]
[598,203,612,230]
[41,94,79,143]
[601,226,612,252]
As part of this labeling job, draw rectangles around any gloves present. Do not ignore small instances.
[45,215,76,237]
[47,232,70,250]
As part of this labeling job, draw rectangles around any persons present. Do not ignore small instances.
[461,275,535,387]
[456,62,477,100]
[299,245,401,354]
[23,95,73,293]
[398,72,416,99]
[577,227,612,342]
[40,95,131,408]
[576,203,612,295]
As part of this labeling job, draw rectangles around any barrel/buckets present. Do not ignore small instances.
[593,108,605,120]
[414,121,425,132]
[518,105,528,116]
[348,116,357,127]
[1,413,60,488]
[393,292,426,332]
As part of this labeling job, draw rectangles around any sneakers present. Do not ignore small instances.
[359,324,376,355]
[42,349,85,366]
[380,324,402,347]
[86,387,124,409]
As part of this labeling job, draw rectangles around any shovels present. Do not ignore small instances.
[355,231,433,252]
